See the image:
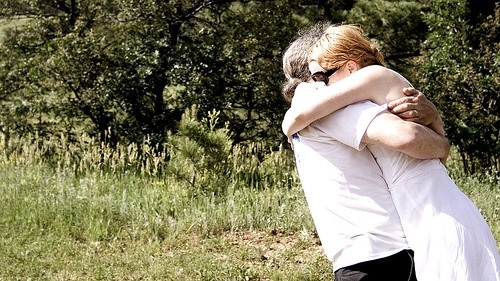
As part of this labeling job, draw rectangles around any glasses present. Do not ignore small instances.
[306,65,346,86]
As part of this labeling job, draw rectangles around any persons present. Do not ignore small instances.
[282,20,449,281]
[283,23,499,281]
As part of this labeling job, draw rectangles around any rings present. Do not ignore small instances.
[413,110,419,116]
